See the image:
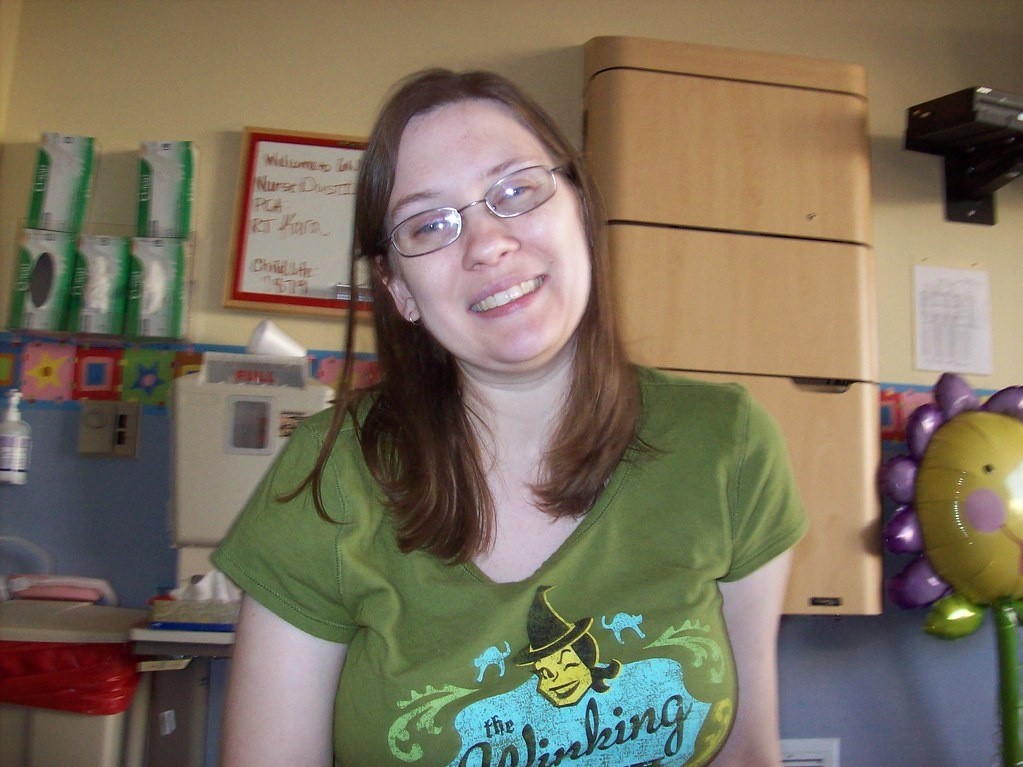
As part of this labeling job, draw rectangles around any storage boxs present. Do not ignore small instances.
[10,130,198,340]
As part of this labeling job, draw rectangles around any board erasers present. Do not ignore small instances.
[336,283,374,302]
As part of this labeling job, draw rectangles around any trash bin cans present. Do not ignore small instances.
[0,598,151,767]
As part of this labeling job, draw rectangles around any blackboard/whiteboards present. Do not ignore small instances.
[222,126,374,319]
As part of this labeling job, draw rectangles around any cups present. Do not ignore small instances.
[245,319,307,358]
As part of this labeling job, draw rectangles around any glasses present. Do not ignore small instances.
[378,165,563,257]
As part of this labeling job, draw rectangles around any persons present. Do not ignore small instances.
[210,66,811,767]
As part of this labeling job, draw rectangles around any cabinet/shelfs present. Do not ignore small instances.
[573,33,884,620]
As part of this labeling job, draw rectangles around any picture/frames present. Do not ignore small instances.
[220,124,379,323]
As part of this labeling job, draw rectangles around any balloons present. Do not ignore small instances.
[878,373,1023,767]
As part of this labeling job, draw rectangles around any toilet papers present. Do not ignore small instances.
[243,318,308,356]
